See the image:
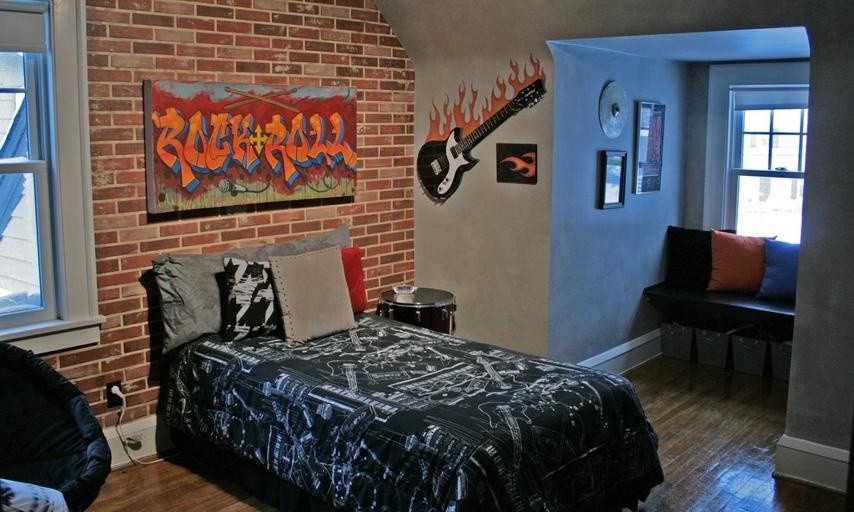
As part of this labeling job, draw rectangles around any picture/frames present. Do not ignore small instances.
[633,99,666,196]
[597,150,628,209]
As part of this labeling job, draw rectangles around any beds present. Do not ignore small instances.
[144,225,645,512]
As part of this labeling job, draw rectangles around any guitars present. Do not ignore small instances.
[416,79,547,202]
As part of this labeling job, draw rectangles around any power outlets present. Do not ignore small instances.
[100,379,125,406]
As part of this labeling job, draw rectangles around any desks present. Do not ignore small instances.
[643,279,816,399]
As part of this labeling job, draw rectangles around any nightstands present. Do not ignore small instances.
[378,282,457,339]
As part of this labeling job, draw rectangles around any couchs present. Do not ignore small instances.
[2,340,112,511]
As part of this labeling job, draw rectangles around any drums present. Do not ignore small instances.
[376,288,457,335]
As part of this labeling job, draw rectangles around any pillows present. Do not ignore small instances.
[707,229,778,294]
[269,246,359,344]
[340,248,369,313]
[760,240,807,298]
[219,257,289,339]
[665,226,735,297]
[153,227,350,356]
